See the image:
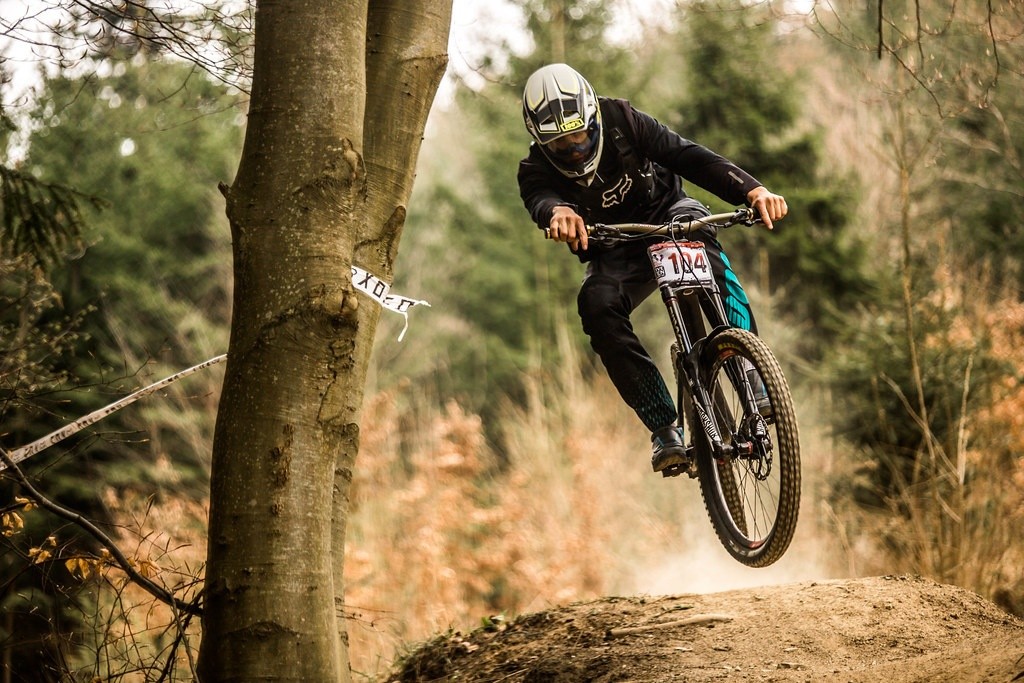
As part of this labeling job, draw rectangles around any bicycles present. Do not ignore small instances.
[543,203,802,569]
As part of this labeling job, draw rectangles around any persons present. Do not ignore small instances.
[516,63,790,475]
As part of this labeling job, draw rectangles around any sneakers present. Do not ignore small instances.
[650,428,689,470]
[747,396,776,424]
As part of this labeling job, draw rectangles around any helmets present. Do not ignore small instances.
[522,64,605,178]
